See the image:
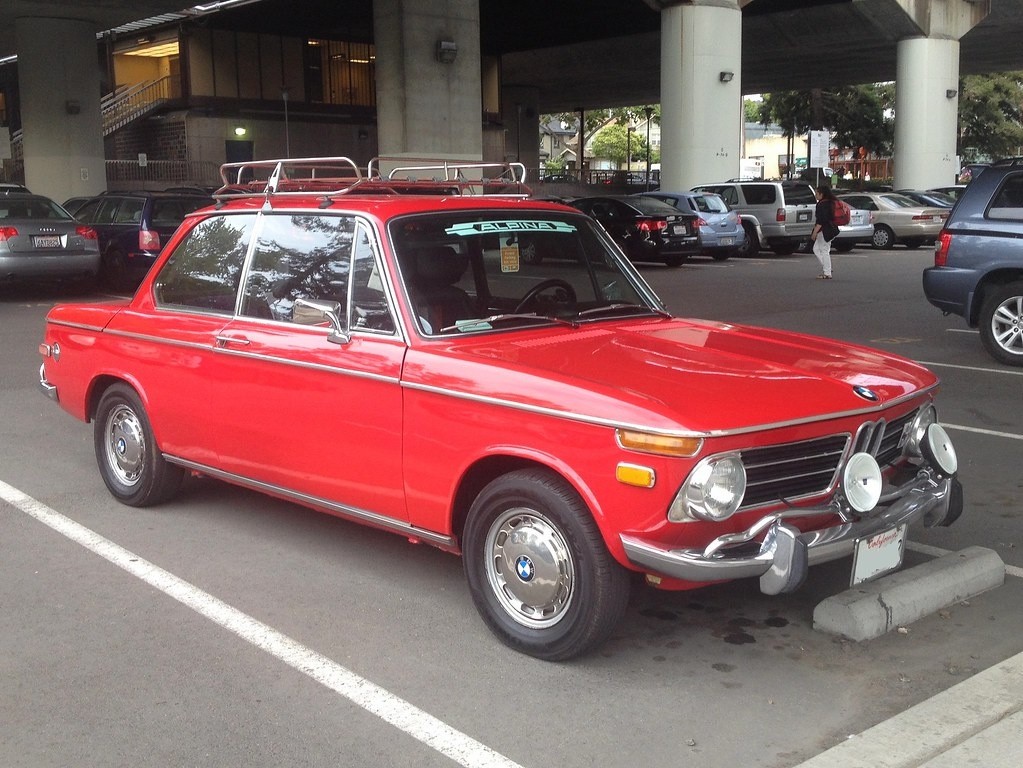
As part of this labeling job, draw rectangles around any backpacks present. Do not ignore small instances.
[818,198,851,226]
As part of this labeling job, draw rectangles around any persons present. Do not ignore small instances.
[845,171,853,179]
[865,172,871,181]
[811,186,840,280]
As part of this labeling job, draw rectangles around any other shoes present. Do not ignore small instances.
[815,273,832,279]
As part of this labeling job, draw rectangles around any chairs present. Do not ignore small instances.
[131,209,142,222]
[7,204,29,219]
[415,233,473,326]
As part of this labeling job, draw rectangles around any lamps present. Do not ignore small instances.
[720,71,734,82]
[435,40,457,64]
[235,125,247,136]
[946,89,957,98]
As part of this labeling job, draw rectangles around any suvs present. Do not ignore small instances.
[690,180,818,259]
[922,156,1023,367]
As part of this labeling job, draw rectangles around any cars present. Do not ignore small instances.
[837,192,950,250]
[73,190,216,293]
[524,193,703,273]
[898,183,970,244]
[631,190,745,260]
[1,190,100,298]
[37,154,965,662]
[796,201,874,254]
